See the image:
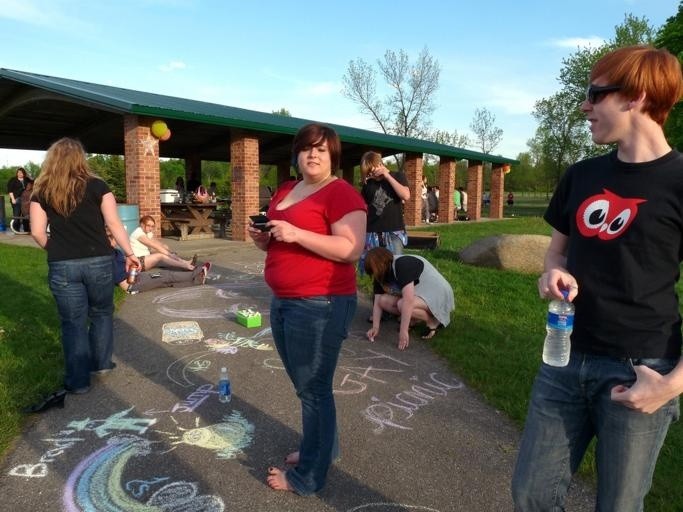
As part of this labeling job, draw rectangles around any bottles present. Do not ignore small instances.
[218,367,231,403]
[540,289,575,369]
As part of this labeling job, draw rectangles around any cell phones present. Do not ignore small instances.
[250,215,274,232]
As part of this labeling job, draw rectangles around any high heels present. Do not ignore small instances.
[20,389,68,413]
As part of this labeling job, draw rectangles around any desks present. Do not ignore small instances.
[159,201,225,234]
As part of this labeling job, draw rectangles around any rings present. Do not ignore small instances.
[569,286,578,289]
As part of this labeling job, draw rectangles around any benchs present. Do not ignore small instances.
[209,214,230,237]
[406,230,441,250]
[7,216,31,236]
[161,215,195,240]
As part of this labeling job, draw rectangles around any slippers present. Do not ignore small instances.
[200,262,211,285]
[421,323,442,342]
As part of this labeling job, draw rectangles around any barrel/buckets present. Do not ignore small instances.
[118,203,140,237]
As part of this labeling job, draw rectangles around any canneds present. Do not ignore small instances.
[127,265,138,284]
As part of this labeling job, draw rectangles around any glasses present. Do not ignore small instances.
[587,83,624,106]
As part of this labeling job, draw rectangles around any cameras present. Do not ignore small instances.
[366,171,376,180]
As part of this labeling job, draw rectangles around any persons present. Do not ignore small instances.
[482,191,487,208]
[264,186,274,197]
[101,222,139,294]
[19,181,34,215]
[246,121,366,494]
[355,149,410,324]
[507,190,515,217]
[126,217,197,272]
[421,176,468,223]
[186,174,200,192]
[6,167,31,234]
[207,182,218,195]
[363,247,455,351]
[26,136,142,398]
[175,176,185,192]
[508,47,683,512]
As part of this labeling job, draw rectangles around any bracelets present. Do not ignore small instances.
[125,252,136,259]
